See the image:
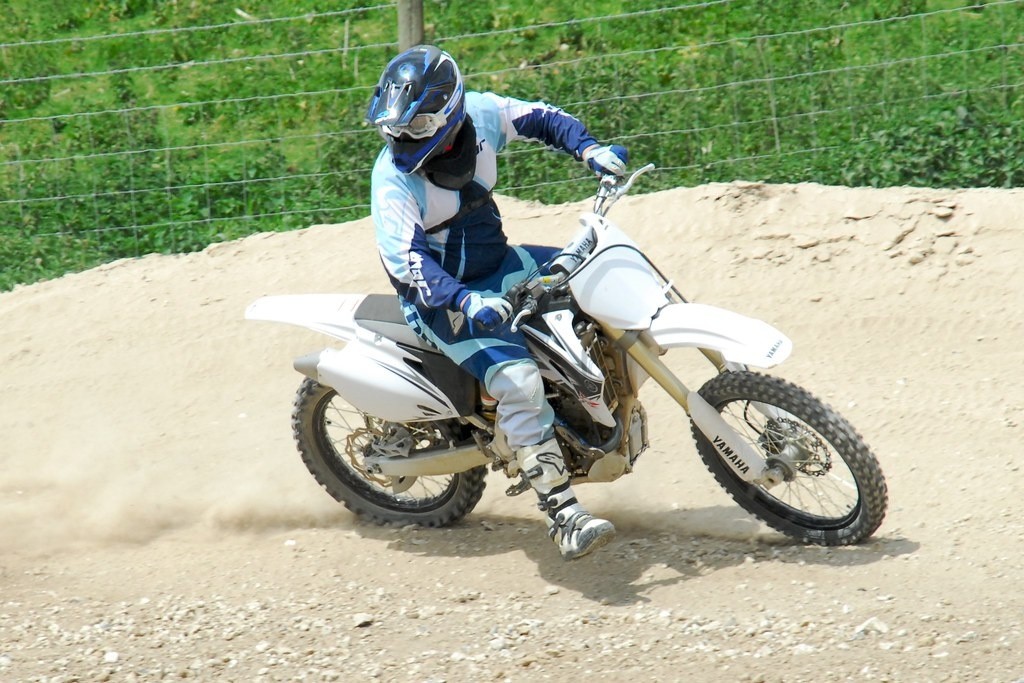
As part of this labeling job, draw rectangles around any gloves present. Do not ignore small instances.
[462,293,513,336]
[583,144,628,181]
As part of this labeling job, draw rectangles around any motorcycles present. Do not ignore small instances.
[242,141,888,550]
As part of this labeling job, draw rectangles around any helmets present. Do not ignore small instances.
[363,45,466,175]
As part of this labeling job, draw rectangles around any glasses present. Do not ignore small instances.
[383,69,463,140]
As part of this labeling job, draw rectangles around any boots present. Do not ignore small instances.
[516,437,616,561]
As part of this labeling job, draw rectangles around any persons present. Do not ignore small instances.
[364,44,628,562]
